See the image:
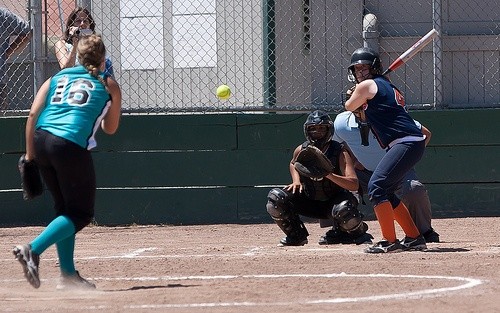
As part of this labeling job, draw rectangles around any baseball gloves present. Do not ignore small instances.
[294,146,334,179]
[17,154,46,201]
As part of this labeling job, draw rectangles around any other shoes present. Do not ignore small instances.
[424,230,439,243]
[319,229,343,246]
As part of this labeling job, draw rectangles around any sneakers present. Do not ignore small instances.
[364,238,402,254]
[354,234,372,244]
[389,235,427,253]
[57,272,97,291]
[13,243,41,289]
[280,236,308,247]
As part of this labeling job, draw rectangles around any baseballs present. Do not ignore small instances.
[217,85,230,100]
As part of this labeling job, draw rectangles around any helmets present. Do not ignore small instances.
[303,110,334,146]
[347,48,382,84]
[346,85,368,110]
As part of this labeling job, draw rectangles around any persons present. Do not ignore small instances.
[55,6,95,71]
[12,35,122,291]
[265,110,360,247]
[343,47,426,254]
[332,107,439,243]
[0,8,32,111]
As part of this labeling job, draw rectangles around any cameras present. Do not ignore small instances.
[68,27,92,36]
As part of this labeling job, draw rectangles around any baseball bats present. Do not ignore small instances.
[383,28,439,77]
